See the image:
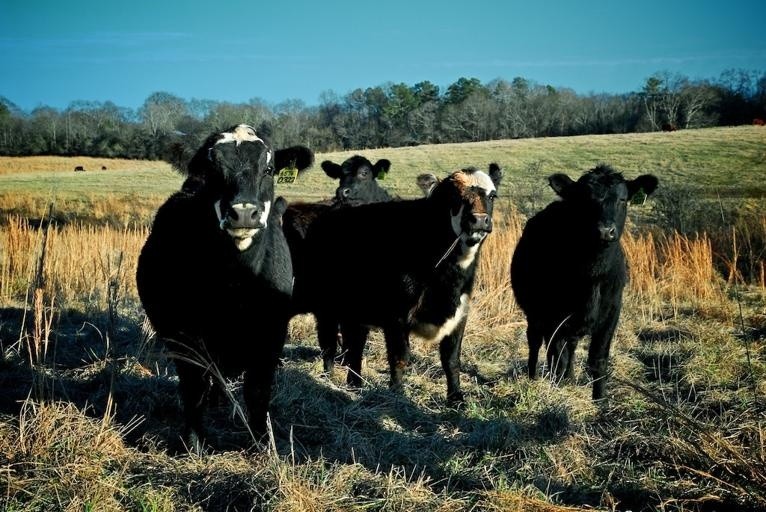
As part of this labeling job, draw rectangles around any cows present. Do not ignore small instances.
[272,155,502,412]
[135,123,316,456]
[509,161,659,412]
